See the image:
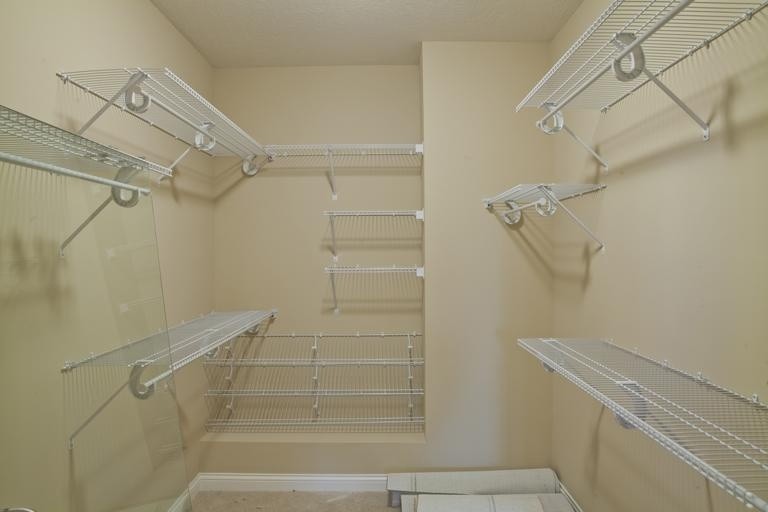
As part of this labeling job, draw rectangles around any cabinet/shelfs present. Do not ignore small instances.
[0,67,423,460]
[483,0,768,511]
